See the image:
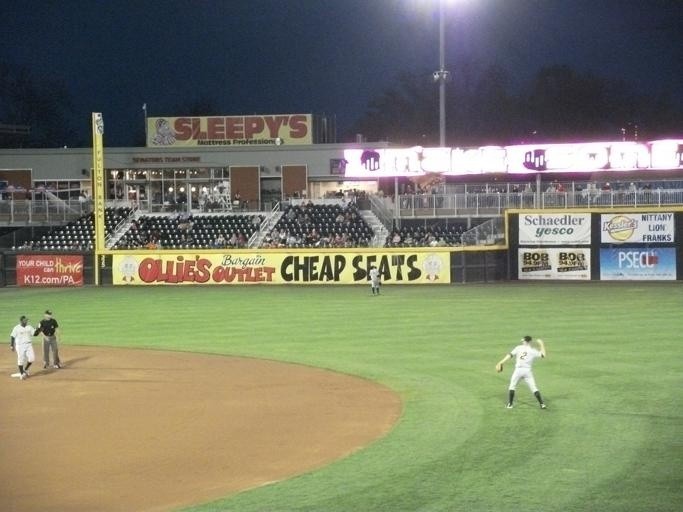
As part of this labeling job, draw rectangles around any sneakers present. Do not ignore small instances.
[504,402,513,408]
[24,368,31,376]
[43,363,49,369]
[53,363,62,369]
[19,373,25,380]
[540,403,546,409]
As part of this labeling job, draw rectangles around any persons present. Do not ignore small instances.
[502,177,682,212]
[8,315,43,380]
[144,236,158,249]
[175,207,193,234]
[0,179,216,213]
[36,309,62,370]
[368,264,380,296]
[211,179,504,248]
[494,335,548,410]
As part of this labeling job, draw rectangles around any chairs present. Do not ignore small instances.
[8,197,493,252]
